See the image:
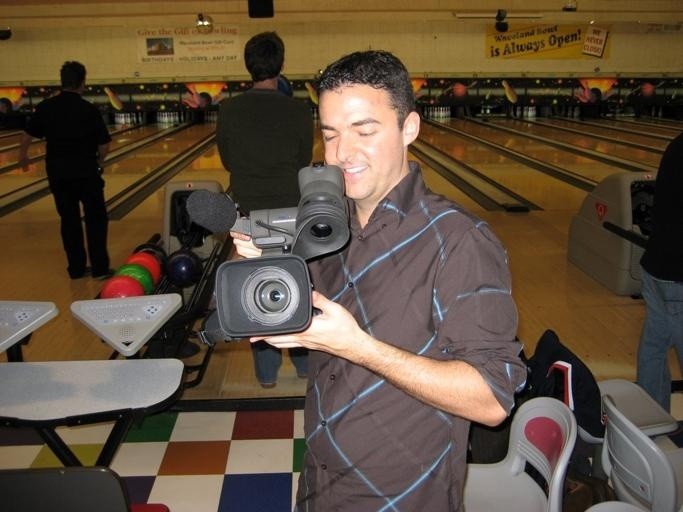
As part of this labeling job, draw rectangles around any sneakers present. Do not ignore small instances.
[92,269,114,280]
[72,266,92,277]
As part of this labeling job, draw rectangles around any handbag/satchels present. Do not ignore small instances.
[544,475,617,512]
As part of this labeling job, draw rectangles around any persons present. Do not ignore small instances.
[17,59,116,283]
[212,30,316,389]
[226,47,529,512]
[635,131,680,438]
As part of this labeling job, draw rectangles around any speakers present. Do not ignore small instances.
[248,0,274,18]
[0,29,12,40]
[495,22,509,32]
